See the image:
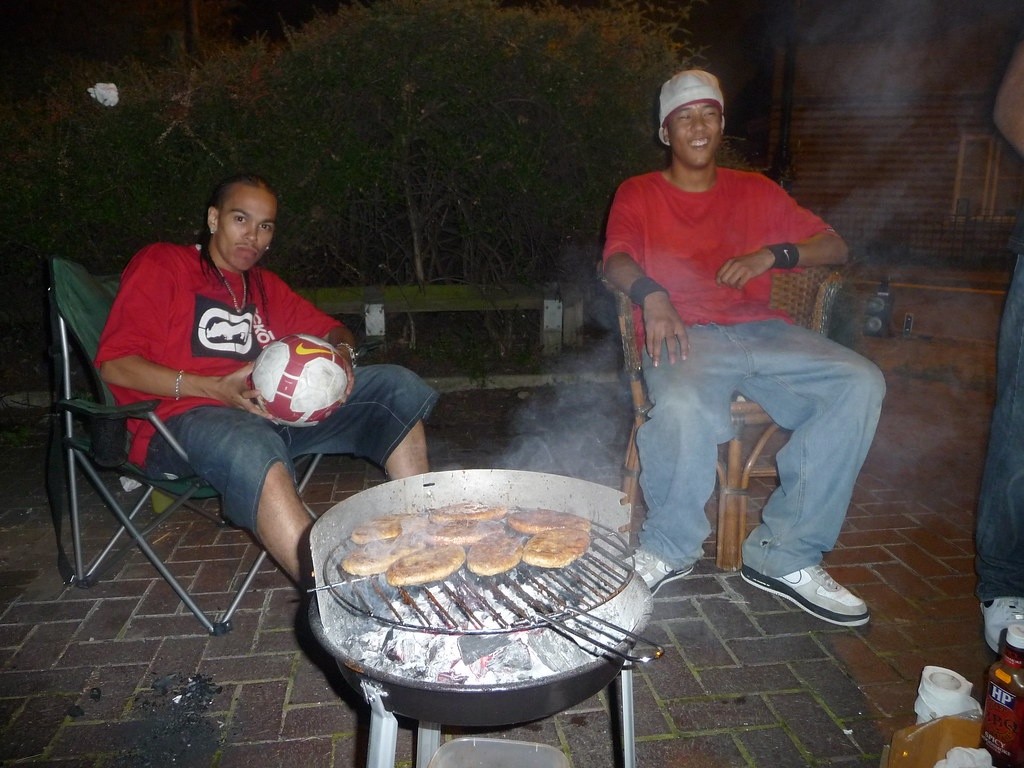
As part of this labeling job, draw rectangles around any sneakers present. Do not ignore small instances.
[980,598,1023,653]
[740,546,869,626]
[621,551,694,596]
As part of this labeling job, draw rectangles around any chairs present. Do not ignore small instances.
[593,265,849,574]
[52,255,318,637]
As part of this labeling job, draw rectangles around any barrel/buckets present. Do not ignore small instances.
[425,736,570,768]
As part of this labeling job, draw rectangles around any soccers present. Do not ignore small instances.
[250,333,354,428]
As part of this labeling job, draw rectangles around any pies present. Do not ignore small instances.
[339,502,590,585]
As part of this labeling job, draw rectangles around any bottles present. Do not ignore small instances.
[981,645,1024,768]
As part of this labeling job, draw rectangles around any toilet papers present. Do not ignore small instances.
[913,665,983,727]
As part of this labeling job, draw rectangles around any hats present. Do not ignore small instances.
[659,69,724,145]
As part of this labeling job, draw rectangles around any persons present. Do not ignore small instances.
[601,68,886,627]
[92,175,439,598]
[974,34,1024,653]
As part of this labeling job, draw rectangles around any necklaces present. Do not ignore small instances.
[217,264,247,314]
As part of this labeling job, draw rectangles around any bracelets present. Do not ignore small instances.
[337,342,357,368]
[175,370,184,400]
[631,276,669,306]
[764,242,800,268]
